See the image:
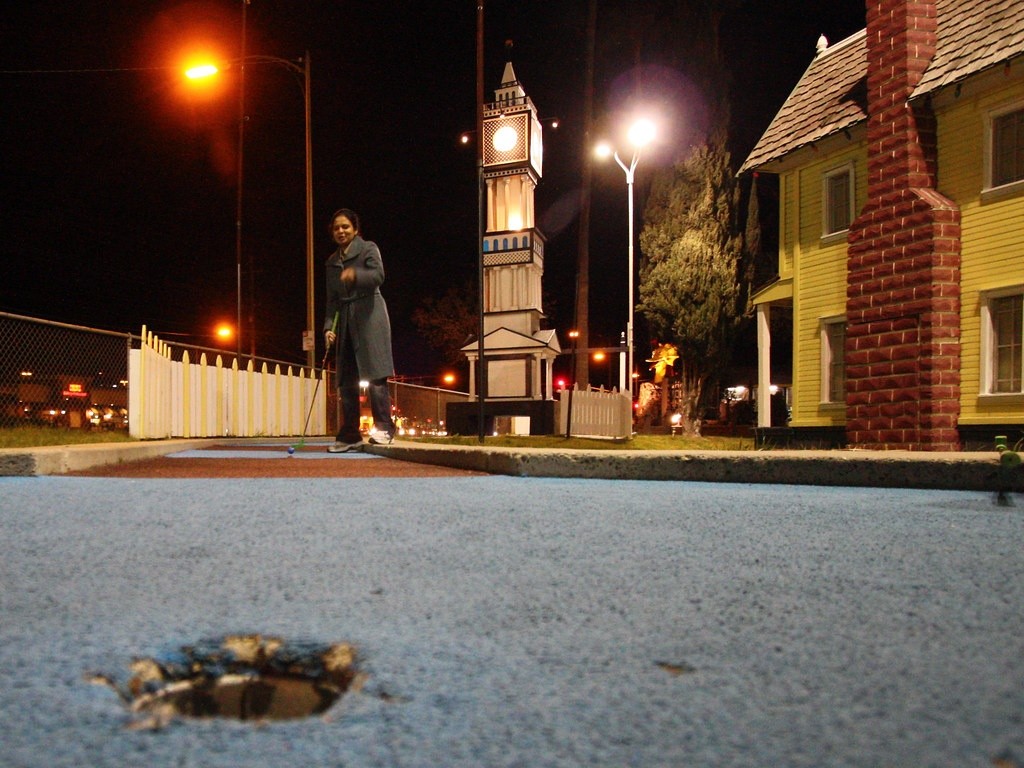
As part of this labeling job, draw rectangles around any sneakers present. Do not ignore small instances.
[368,431,394,445]
[327,439,363,453]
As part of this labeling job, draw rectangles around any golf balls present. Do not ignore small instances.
[287,447,295,454]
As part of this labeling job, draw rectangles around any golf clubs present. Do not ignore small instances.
[288,311,339,448]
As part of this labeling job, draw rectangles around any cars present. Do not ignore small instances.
[33,402,129,431]
[0,394,33,423]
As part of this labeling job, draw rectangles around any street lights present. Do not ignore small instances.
[182,49,315,378]
[593,118,658,440]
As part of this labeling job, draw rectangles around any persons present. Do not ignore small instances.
[322,208,395,454]
